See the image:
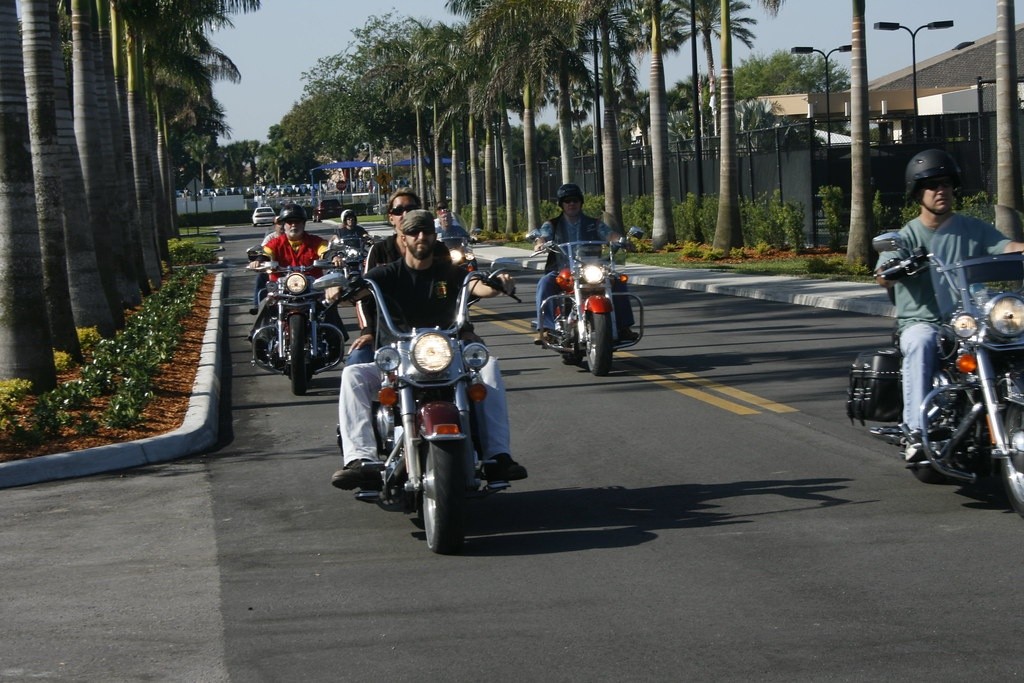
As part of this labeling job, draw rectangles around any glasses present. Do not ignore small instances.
[560,195,582,204]
[437,206,448,210]
[388,204,422,216]
[345,216,355,220]
[402,226,437,238]
[921,178,955,190]
[283,218,304,224]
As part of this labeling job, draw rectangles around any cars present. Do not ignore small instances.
[252,206,276,227]
[311,198,343,223]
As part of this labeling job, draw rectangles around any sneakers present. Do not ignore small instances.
[899,429,924,463]
[331,457,383,491]
[485,453,527,483]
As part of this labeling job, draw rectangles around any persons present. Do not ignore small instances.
[249,204,349,363]
[325,186,527,487]
[874,149,1024,463]
[531,183,639,342]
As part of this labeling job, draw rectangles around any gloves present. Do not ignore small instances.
[880,259,905,281]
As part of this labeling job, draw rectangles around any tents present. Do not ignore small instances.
[308,156,452,203]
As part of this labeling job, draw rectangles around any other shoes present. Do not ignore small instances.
[255,334,269,348]
[533,329,551,345]
[617,328,638,341]
[250,308,259,315]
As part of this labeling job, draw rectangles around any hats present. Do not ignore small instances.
[398,210,437,236]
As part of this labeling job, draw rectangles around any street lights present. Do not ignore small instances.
[790,44,852,147]
[873,19,954,146]
[362,141,374,179]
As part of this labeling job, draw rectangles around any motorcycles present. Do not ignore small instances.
[434,211,482,308]
[245,245,345,398]
[844,233,1024,522]
[334,235,383,306]
[524,226,643,376]
[313,257,525,557]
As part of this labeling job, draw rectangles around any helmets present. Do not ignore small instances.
[278,204,308,223]
[556,184,583,200]
[341,209,357,225]
[905,150,962,196]
